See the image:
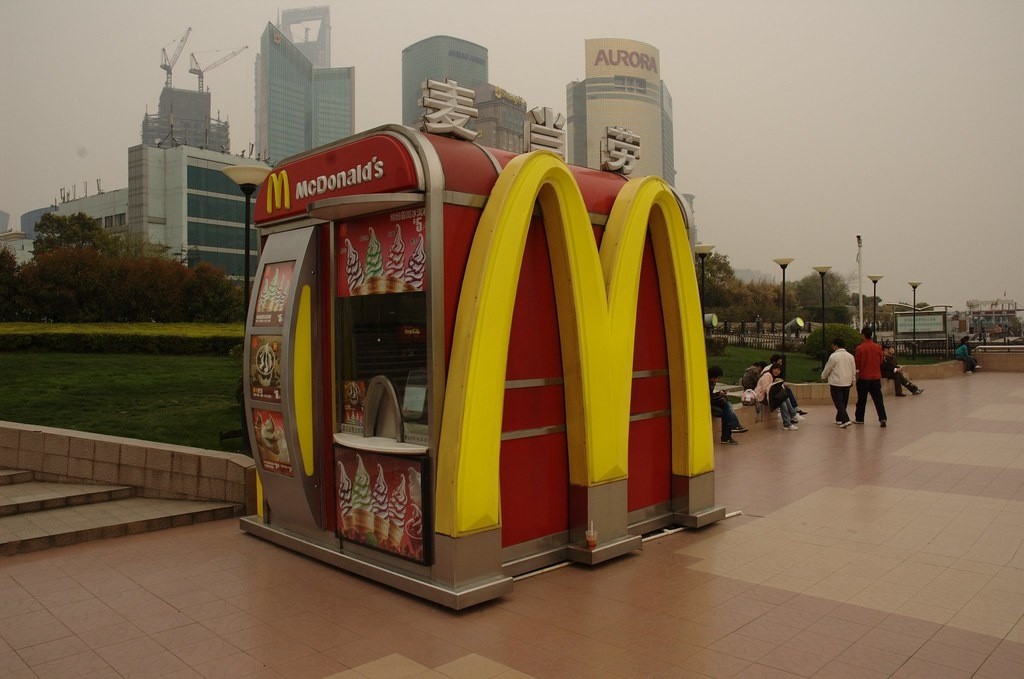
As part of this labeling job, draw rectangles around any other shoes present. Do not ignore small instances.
[797,410,807,415]
[790,420,798,423]
[851,419,864,424]
[880,420,886,428]
[913,387,924,395]
[895,393,906,397]
[731,425,749,433]
[974,365,982,368]
[966,371,972,373]
[840,421,853,428]
[782,424,798,430]
[720,437,738,445]
[792,413,806,421]
[835,421,842,424]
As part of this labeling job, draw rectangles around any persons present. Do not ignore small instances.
[760,355,807,424]
[708,365,749,445]
[819,338,857,428]
[852,327,888,427]
[880,346,924,396]
[742,361,767,390]
[994,324,1008,332]
[755,364,804,431]
[955,336,982,374]
[754,315,763,333]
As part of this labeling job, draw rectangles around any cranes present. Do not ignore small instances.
[190,45,250,92]
[159,26,193,89]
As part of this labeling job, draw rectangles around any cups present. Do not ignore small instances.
[255,345,275,387]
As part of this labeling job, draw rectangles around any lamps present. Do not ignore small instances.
[785,317,804,338]
[705,313,718,335]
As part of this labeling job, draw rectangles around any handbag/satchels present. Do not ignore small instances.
[741,389,757,406]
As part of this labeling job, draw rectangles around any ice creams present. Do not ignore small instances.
[345,411,363,426]
[253,413,290,466]
[259,268,290,312]
[256,342,276,387]
[338,453,423,560]
[345,223,426,295]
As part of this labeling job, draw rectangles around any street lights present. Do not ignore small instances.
[694,244,716,329]
[907,280,923,361]
[867,275,884,341]
[773,258,795,377]
[811,266,833,371]
[222,164,272,447]
